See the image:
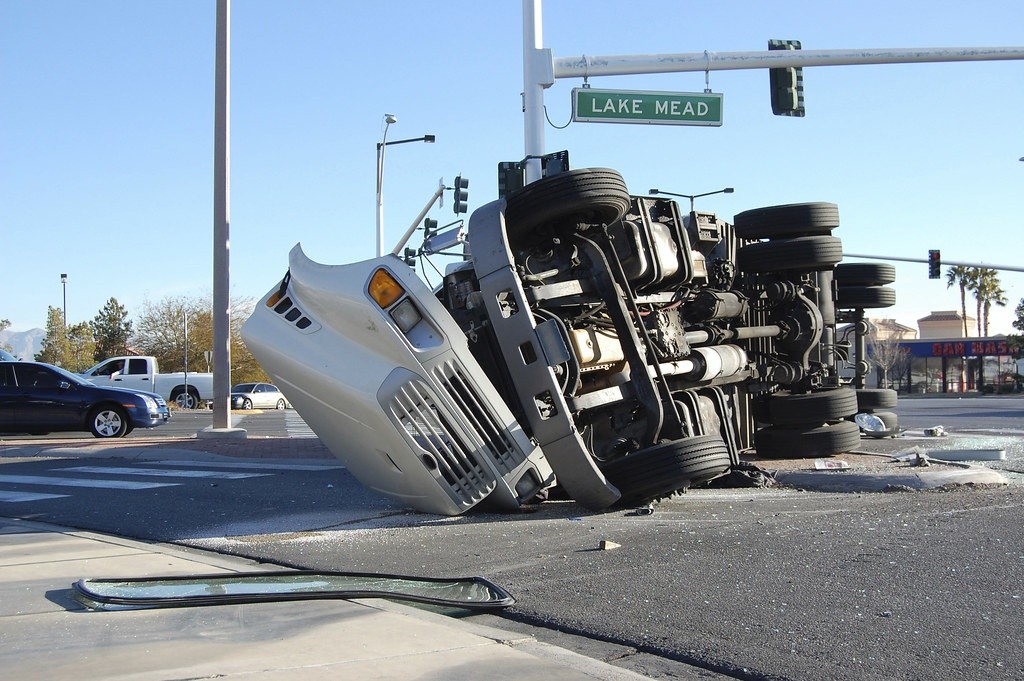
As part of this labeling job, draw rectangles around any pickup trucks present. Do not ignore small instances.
[71,356,214,410]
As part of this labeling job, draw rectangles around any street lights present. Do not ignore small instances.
[376,114,436,257]
[61,274,68,326]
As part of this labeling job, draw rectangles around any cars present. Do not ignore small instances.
[0,361,172,438]
[0,349,24,361]
[230,382,291,410]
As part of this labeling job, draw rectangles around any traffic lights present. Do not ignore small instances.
[928,250,941,279]
[404,246,416,273]
[453,172,469,217]
[424,218,438,242]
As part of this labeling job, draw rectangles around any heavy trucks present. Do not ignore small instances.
[240,170,900,517]
